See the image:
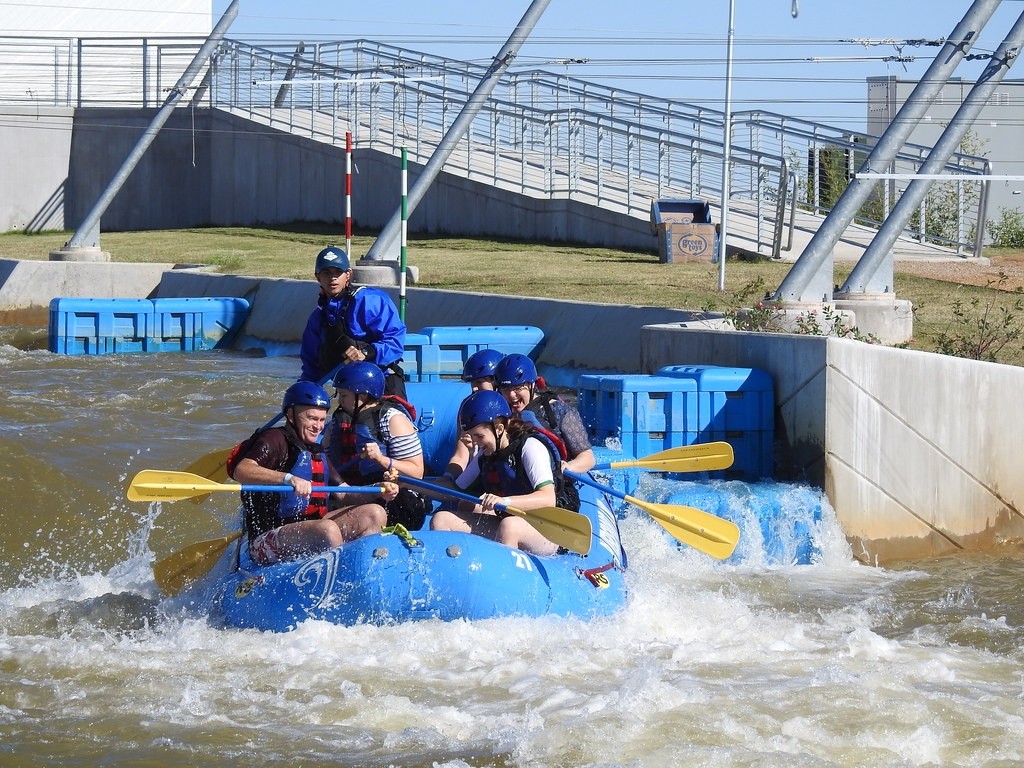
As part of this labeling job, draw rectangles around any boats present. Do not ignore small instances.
[220,454,631,636]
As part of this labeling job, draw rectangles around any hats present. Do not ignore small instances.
[315,247,350,273]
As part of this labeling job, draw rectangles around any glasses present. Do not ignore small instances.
[499,386,525,394]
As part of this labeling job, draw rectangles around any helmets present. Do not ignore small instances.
[333,361,385,400]
[282,381,331,417]
[494,353,538,390]
[461,349,507,382]
[459,390,512,431]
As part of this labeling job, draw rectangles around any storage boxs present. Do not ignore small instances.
[651,198,718,264]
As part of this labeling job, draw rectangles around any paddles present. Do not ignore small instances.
[183,358,353,505]
[388,467,593,557]
[126,467,388,503]
[422,440,736,484]
[153,451,369,599]
[563,468,741,561]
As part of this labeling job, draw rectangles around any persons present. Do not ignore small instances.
[383,349,596,555]
[233,246,425,565]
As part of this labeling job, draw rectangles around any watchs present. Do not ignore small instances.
[362,349,368,356]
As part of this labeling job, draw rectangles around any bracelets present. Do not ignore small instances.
[503,497,511,505]
[283,473,293,485]
[386,458,392,470]
